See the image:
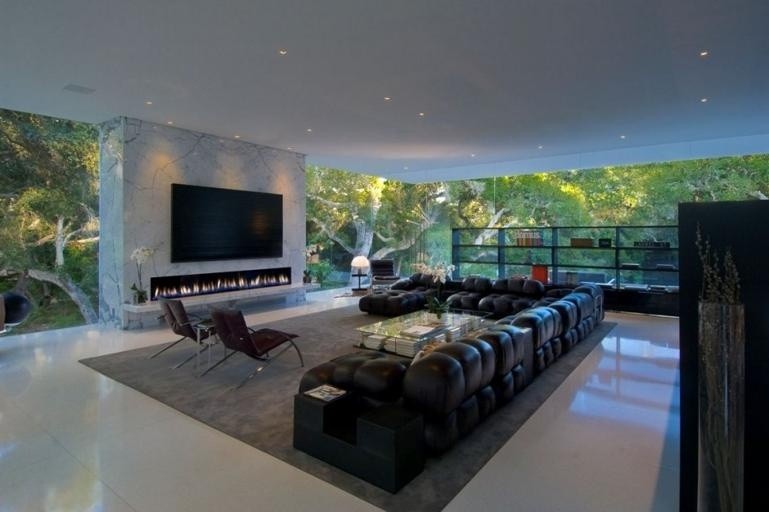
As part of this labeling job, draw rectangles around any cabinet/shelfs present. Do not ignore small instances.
[450,226,681,318]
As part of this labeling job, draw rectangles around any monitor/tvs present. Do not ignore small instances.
[170,183,283,263]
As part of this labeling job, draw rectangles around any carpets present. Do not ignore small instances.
[79,303,621,512]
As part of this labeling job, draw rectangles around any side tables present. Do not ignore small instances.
[294,385,437,494]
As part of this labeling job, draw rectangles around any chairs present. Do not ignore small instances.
[150,297,304,391]
[370,259,400,292]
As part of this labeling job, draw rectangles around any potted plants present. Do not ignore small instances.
[691,238,748,510]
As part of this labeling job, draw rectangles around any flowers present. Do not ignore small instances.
[131,247,154,292]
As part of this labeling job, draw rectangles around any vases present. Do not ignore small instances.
[132,289,148,305]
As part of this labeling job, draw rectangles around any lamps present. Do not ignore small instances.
[351,256,370,295]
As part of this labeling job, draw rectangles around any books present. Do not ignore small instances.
[515,230,544,247]
[624,283,672,294]
[303,383,345,402]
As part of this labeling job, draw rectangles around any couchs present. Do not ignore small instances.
[478,283,604,403]
[360,271,577,312]
[301,337,496,460]
